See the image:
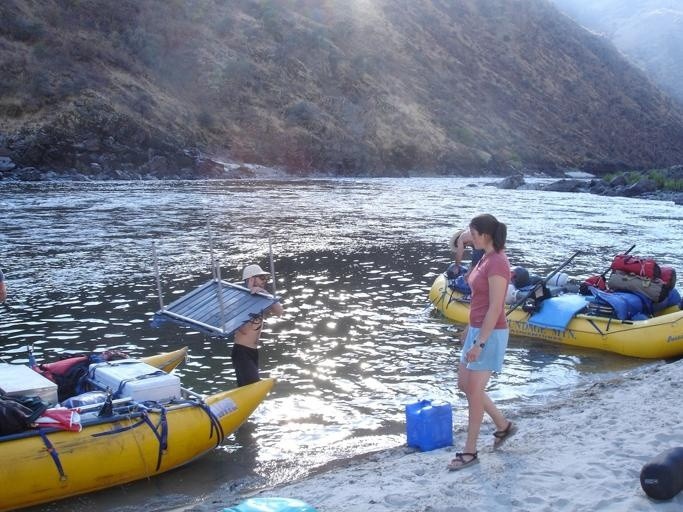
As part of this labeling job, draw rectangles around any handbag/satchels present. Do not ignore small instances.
[608,254,676,303]
[54,359,91,402]
[0,389,50,436]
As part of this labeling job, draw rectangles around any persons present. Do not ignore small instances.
[449,230,484,283]
[231,264,283,387]
[0,271,6,302]
[447,213,518,471]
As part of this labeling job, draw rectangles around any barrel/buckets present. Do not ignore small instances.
[406,399,453,451]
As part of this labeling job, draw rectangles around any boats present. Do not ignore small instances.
[0,346,276,511]
[428,270,683,359]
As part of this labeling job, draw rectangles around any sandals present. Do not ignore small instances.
[493,421,518,449]
[447,450,480,471]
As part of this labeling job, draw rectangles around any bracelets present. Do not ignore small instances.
[473,340,485,348]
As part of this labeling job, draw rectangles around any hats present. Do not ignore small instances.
[449,230,466,253]
[242,264,271,281]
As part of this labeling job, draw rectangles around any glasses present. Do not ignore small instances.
[253,275,270,280]
